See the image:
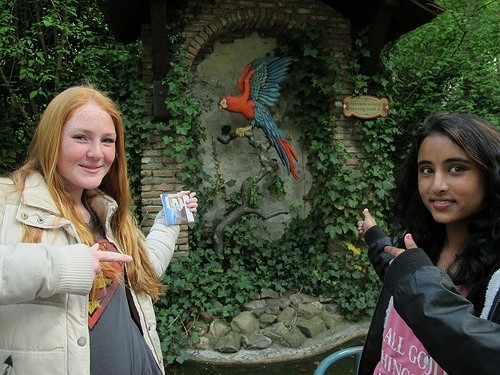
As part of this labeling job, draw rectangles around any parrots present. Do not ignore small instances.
[217,56,300,181]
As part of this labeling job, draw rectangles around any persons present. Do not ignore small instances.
[357,116,500,375]
[0,88,196,375]
